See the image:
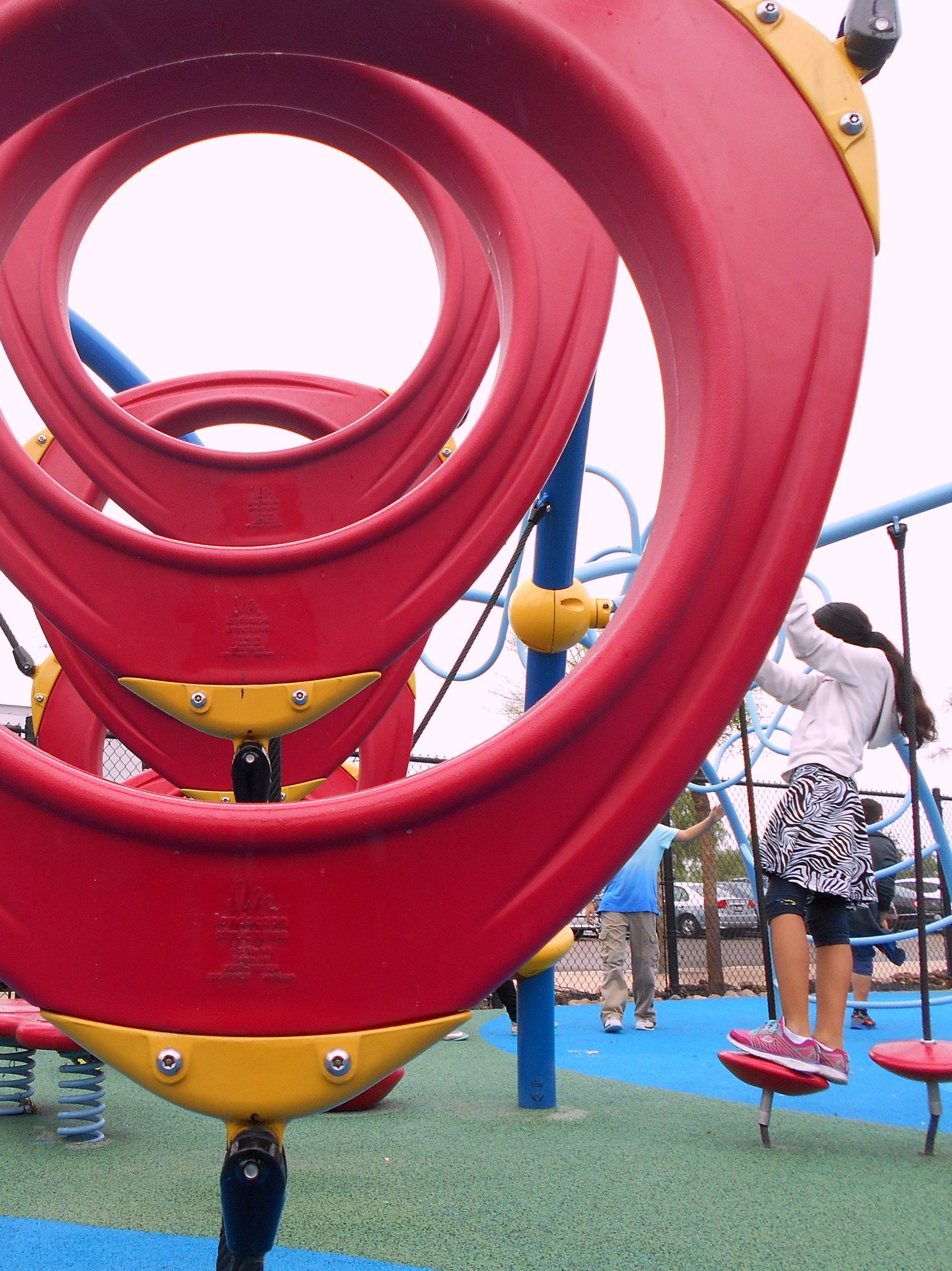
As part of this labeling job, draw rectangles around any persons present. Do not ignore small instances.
[584,803,726,1036]
[441,976,561,1041]
[728,580,938,1086]
[850,795,908,1030]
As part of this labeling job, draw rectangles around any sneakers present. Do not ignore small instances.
[603,1016,623,1032]
[635,1018,655,1031]
[810,1037,850,1085]
[511,1021,559,1036]
[728,1019,822,1076]
[849,1012,878,1031]
[439,1029,469,1041]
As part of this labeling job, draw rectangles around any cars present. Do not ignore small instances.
[569,877,949,940]
